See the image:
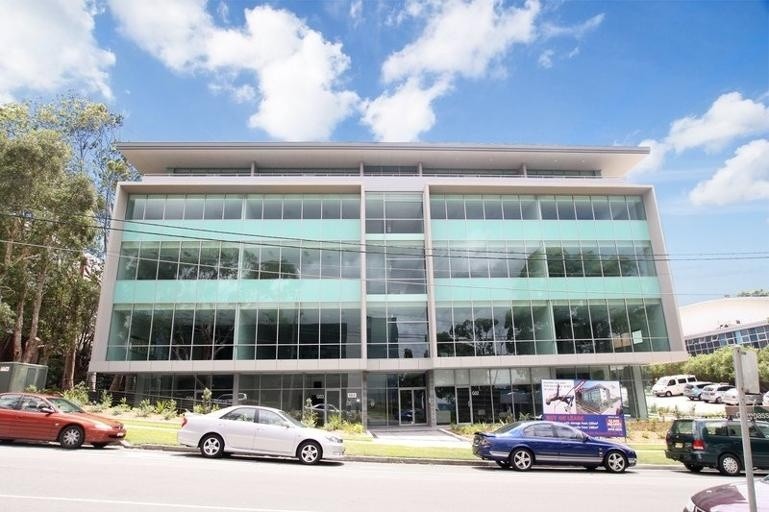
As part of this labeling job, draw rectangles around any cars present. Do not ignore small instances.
[681,473,768,512]
[395,408,425,423]
[683,381,769,406]
[176,404,347,465]
[304,403,343,425]
[0,392,127,449]
[473,419,638,473]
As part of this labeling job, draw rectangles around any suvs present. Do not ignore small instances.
[664,414,769,476]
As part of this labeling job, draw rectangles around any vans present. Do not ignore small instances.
[651,374,697,397]
[212,392,248,408]
